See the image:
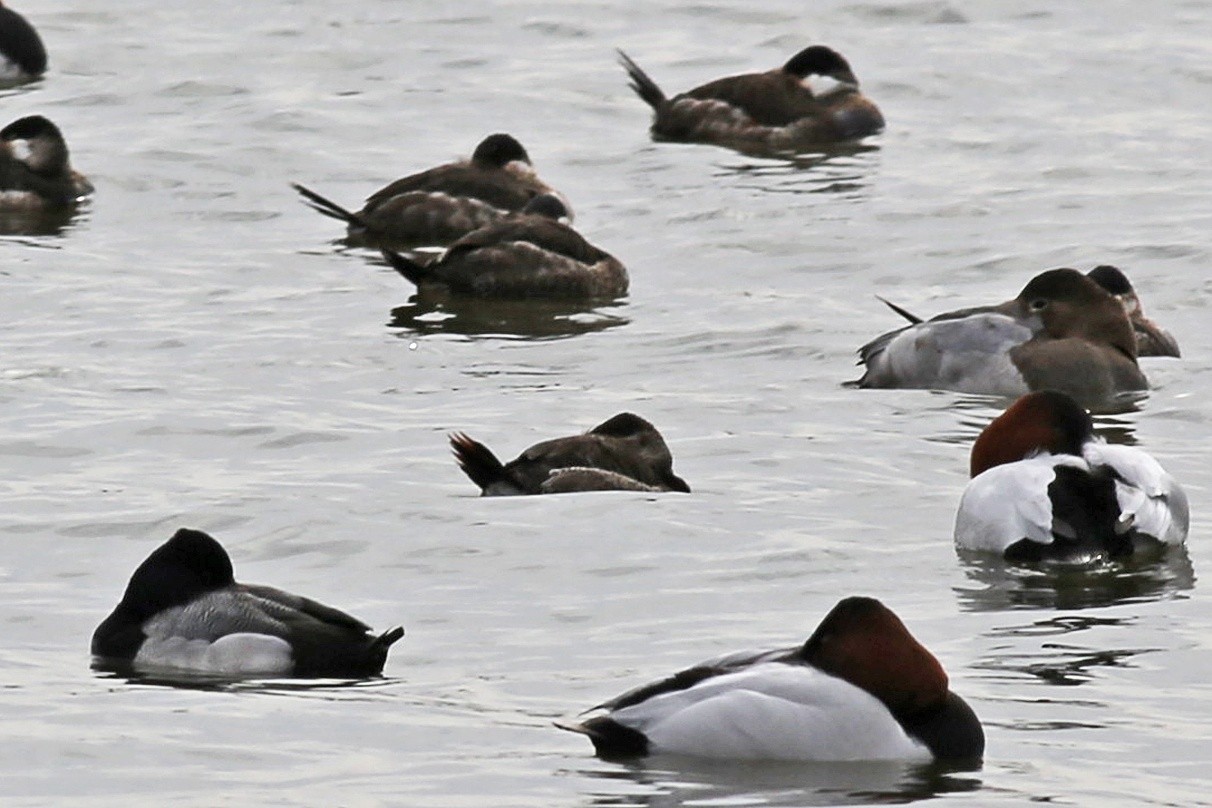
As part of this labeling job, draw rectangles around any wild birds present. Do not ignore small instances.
[549,595,986,767]
[840,265,1189,564]
[0,3,888,297]
[89,528,406,678]
[446,411,692,496]
